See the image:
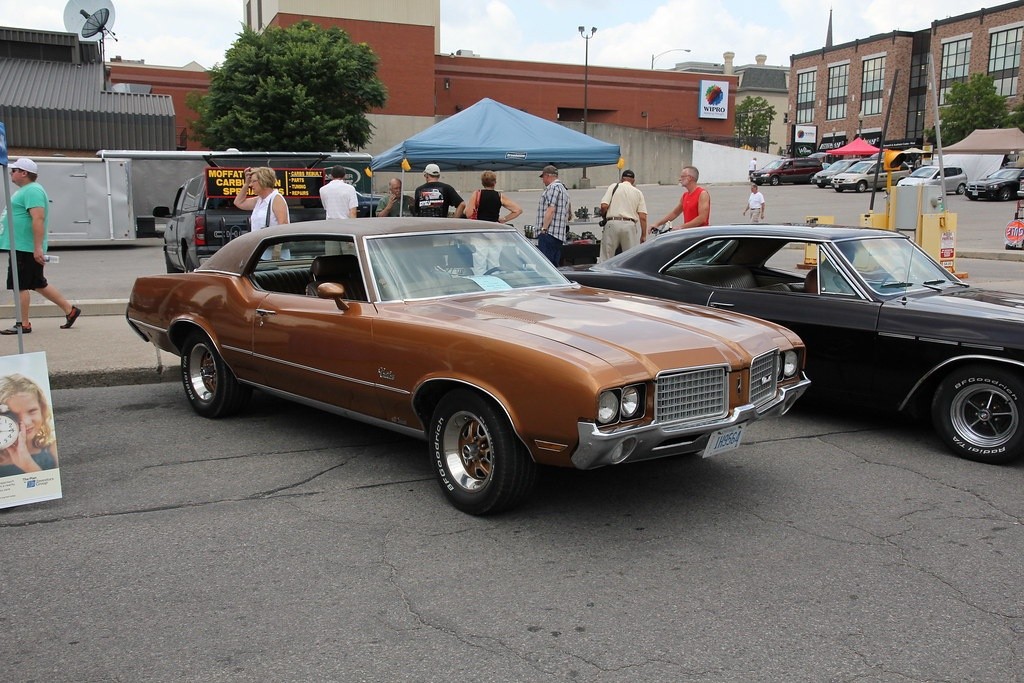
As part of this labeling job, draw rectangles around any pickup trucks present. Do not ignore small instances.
[153,153,331,273]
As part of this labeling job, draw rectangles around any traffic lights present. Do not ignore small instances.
[883,148,907,171]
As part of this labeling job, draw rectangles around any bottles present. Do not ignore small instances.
[42,255,59,263]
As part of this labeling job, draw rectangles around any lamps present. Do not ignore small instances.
[449,53,455,59]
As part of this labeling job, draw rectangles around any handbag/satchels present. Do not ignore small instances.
[261,197,295,250]
[470,189,482,219]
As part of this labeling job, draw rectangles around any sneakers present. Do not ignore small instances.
[0,320,32,334]
[60,306,82,329]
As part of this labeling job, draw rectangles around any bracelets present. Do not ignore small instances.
[541,228,547,231]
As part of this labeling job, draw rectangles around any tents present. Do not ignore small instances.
[370,97,622,218]
[933,127,1024,181]
[0,123,24,354]
[826,137,880,158]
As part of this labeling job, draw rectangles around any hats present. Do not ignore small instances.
[622,169,635,178]
[539,165,559,178]
[8,157,37,175]
[423,163,440,176]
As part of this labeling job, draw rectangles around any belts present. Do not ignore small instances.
[608,217,633,221]
[751,208,760,210]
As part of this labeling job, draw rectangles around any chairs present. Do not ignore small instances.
[306,255,366,302]
[754,283,791,294]
[787,282,805,293]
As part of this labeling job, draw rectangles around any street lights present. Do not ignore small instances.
[579,26,597,188]
[652,49,691,70]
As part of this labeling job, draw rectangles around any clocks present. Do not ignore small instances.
[0,402,21,451]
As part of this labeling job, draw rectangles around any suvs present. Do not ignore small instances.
[749,158,824,186]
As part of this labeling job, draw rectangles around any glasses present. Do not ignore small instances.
[12,169,18,173]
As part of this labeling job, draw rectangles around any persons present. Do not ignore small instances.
[599,168,647,263]
[415,164,466,218]
[319,165,358,255]
[0,372,58,478]
[376,178,417,217]
[535,165,572,271]
[749,158,757,182]
[0,157,81,334]
[743,184,765,223]
[233,166,290,261]
[647,166,710,260]
[803,241,856,294]
[465,170,523,275]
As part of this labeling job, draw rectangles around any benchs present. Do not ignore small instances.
[257,268,314,293]
[664,265,758,288]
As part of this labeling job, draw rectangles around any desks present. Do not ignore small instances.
[536,240,603,265]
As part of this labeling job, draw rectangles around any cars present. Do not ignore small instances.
[356,192,384,217]
[811,153,912,193]
[555,225,1024,464]
[126,217,812,517]
[965,166,1024,202]
[896,166,967,195]
